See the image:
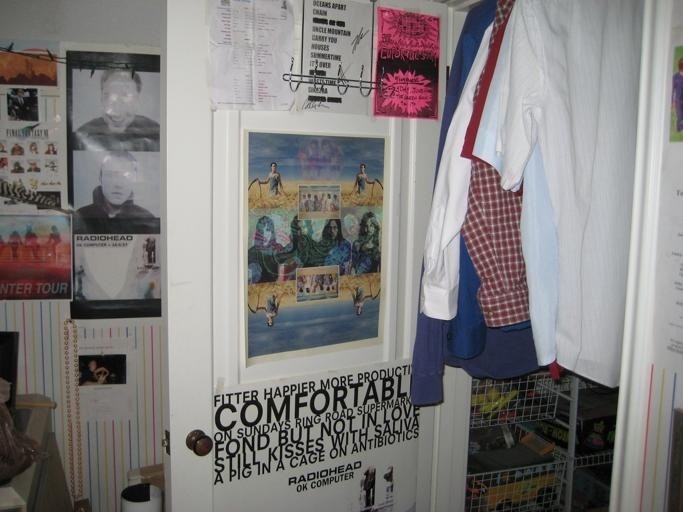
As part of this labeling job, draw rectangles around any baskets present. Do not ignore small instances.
[467,448,569,512]
[470,371,562,430]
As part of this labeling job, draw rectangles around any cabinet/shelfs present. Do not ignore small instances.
[466,369,618,512]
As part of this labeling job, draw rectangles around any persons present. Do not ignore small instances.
[0,70,160,234]
[80,359,109,385]
[248,138,383,328]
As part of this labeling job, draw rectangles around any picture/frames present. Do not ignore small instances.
[211,109,408,391]
